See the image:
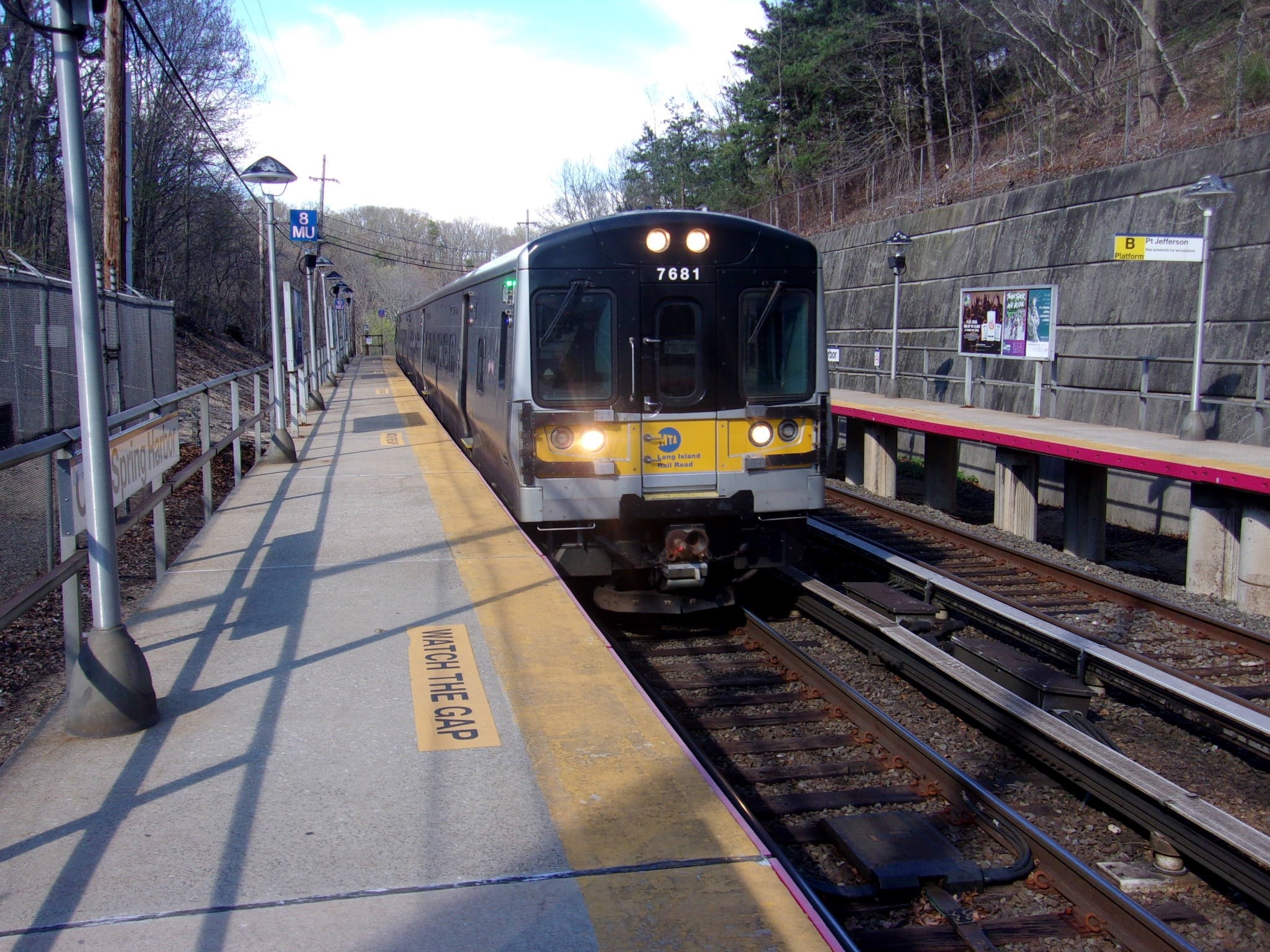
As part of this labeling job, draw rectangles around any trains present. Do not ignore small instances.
[393,210,831,615]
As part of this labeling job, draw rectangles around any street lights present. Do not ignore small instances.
[313,255,355,387]
[884,231,915,399]
[239,155,298,463]
[1179,173,1236,442]
